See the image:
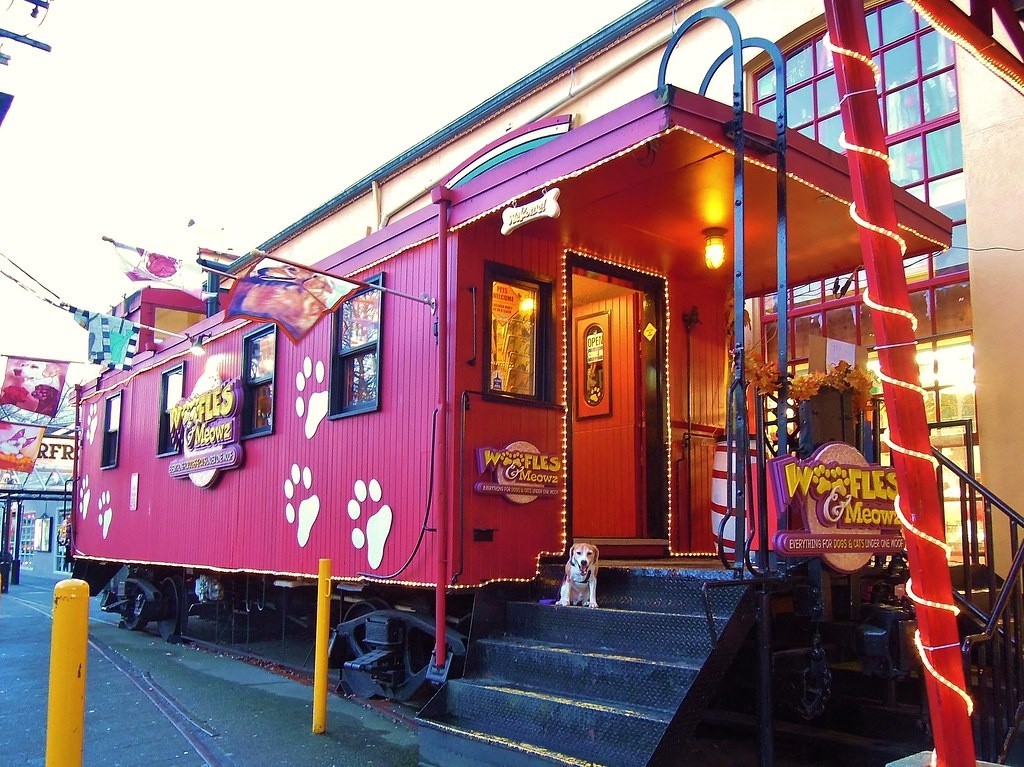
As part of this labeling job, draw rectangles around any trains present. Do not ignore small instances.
[62,7,955,767]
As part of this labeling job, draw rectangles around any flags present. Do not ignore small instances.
[0,421,45,473]
[74,312,141,371]
[222,256,368,346]
[0,357,70,417]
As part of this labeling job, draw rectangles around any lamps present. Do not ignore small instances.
[189,334,210,357]
[702,227,729,270]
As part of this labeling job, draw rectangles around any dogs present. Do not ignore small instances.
[555,543,600,608]
